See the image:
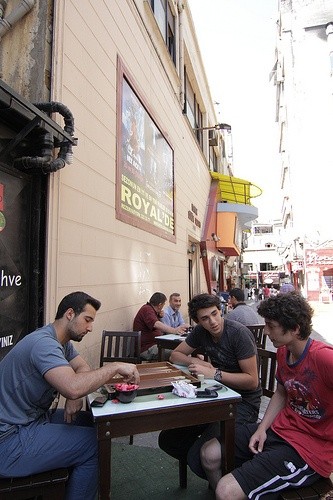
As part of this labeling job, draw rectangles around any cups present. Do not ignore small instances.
[198,375,204,384]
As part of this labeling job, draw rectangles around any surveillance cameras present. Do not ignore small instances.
[211,233,218,242]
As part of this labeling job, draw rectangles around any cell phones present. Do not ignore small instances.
[196,390,218,398]
[205,385,222,391]
[90,396,108,407]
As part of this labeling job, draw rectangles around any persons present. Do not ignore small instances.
[196,289,333,500]
[160,293,189,349]
[157,292,263,491]
[246,277,293,302]
[221,287,265,350]
[132,292,186,361]
[0,291,140,500]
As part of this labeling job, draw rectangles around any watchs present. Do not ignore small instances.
[213,369,222,384]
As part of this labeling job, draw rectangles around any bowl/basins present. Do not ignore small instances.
[115,390,136,403]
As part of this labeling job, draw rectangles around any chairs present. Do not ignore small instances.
[0,469,69,500]
[178,347,278,489]
[100,330,142,368]
[246,325,267,349]
[280,477,333,500]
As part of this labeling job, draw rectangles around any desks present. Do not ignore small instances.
[155,331,192,363]
[86,363,242,500]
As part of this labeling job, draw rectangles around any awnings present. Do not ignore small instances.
[209,170,262,207]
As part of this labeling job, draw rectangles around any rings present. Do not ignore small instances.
[191,372,193,376]
